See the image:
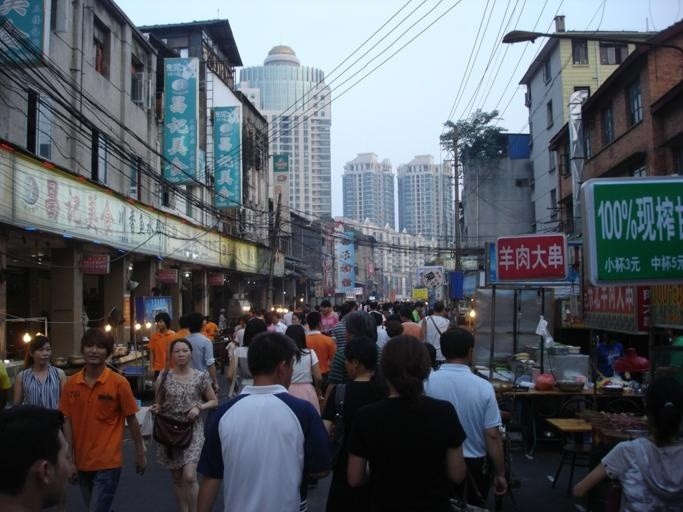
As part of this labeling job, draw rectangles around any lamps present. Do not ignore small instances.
[470,299,476,317]
[135,320,141,329]
[536,289,542,305]
[516,289,523,321]
[146,318,151,328]
[105,321,111,331]
[22,322,32,343]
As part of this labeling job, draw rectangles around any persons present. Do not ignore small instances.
[567,369,683,512]
[0,360,12,410]
[12,336,69,409]
[54,327,148,512]
[0,404,78,511]
[145,293,507,512]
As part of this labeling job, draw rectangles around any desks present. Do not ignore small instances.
[546,418,592,496]
[503,385,645,456]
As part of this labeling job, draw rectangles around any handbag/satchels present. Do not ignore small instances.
[330,384,347,447]
[451,471,490,512]
[153,413,193,450]
